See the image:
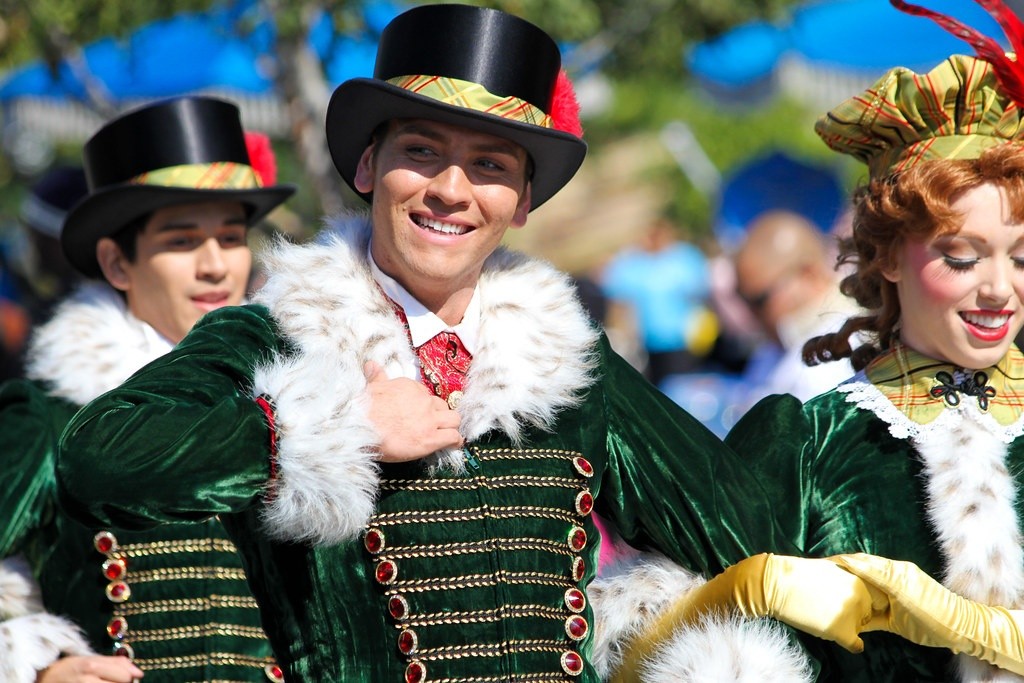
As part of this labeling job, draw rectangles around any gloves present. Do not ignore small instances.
[824,552,1023,677]
[611,552,888,683]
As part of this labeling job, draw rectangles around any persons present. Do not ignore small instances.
[736,213,865,405]
[607,53,1024,683]
[0,96,295,683]
[43,3,897,683]
[602,158,725,379]
[716,55,866,257]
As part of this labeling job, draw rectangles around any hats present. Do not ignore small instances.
[60,93,297,275]
[325,3,588,213]
